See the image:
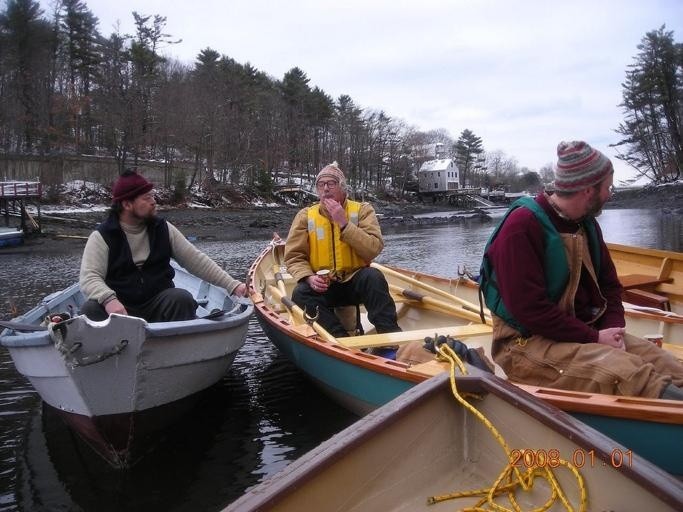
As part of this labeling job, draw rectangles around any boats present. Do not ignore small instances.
[247,235,683,478]
[0,260,253,420]
[601,241,683,319]
[221,347,683,512]
[0,226,26,247]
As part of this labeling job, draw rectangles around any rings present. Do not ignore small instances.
[313,285,317,289]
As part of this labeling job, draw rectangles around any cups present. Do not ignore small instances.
[644,334,663,350]
[315,269,333,290]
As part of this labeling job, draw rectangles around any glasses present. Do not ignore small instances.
[318,181,337,188]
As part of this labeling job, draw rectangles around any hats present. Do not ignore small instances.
[315,160,344,185]
[554,140,614,192]
[111,170,154,201]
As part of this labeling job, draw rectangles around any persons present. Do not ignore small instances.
[79,168,252,325]
[476,137,681,405]
[282,158,406,351]
